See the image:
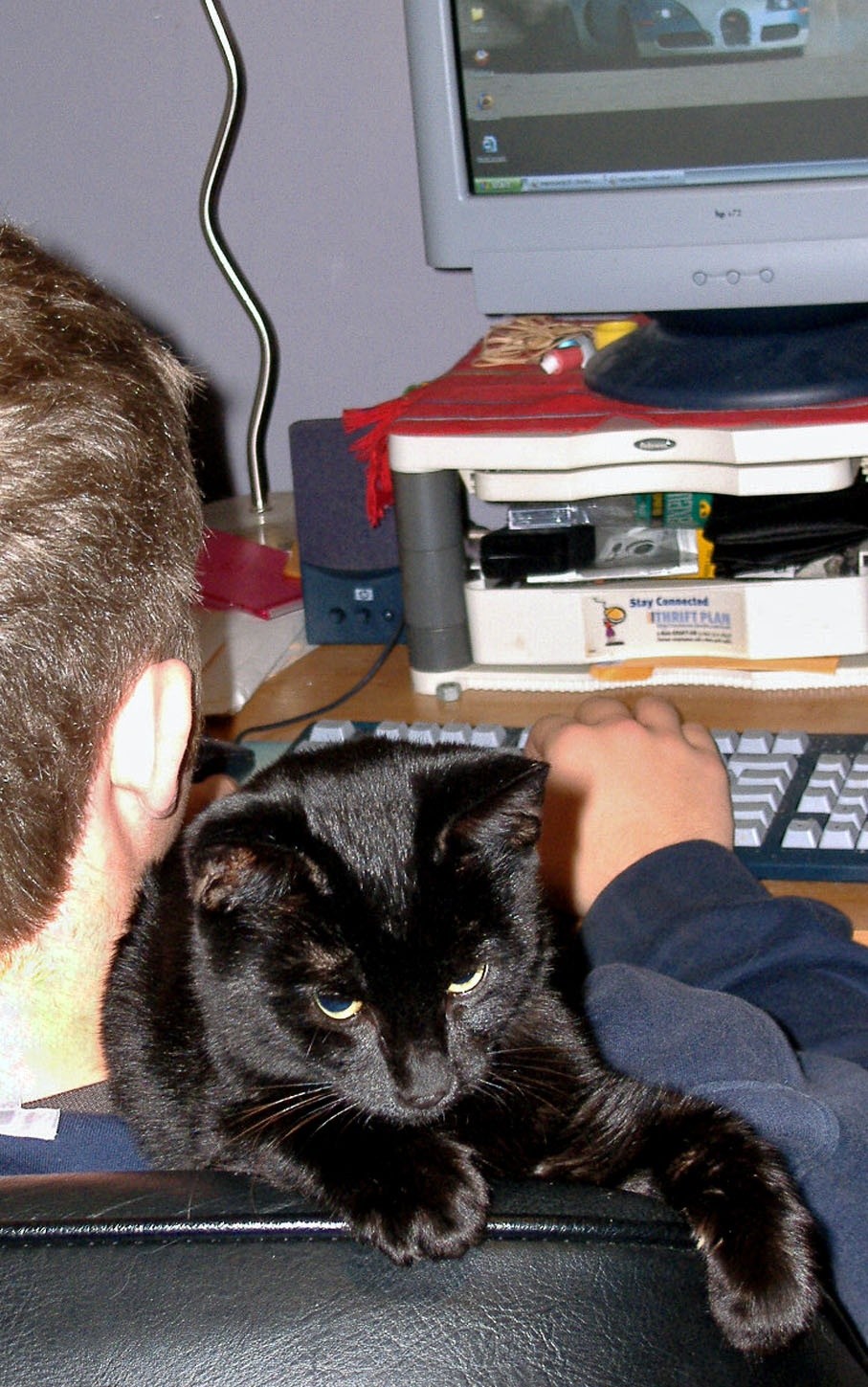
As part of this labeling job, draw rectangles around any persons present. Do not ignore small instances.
[0,219,868,1345]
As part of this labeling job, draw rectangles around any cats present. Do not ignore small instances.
[99,728,826,1367]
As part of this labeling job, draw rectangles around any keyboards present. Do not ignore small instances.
[293,716,868,882]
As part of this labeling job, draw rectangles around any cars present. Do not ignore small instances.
[538,0,813,62]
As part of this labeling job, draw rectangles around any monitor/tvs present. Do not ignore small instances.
[405,0,868,407]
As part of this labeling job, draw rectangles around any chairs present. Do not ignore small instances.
[0,1167,868,1387]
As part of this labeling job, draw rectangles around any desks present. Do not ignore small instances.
[172,637,868,946]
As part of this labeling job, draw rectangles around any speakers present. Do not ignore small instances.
[286,419,409,646]
